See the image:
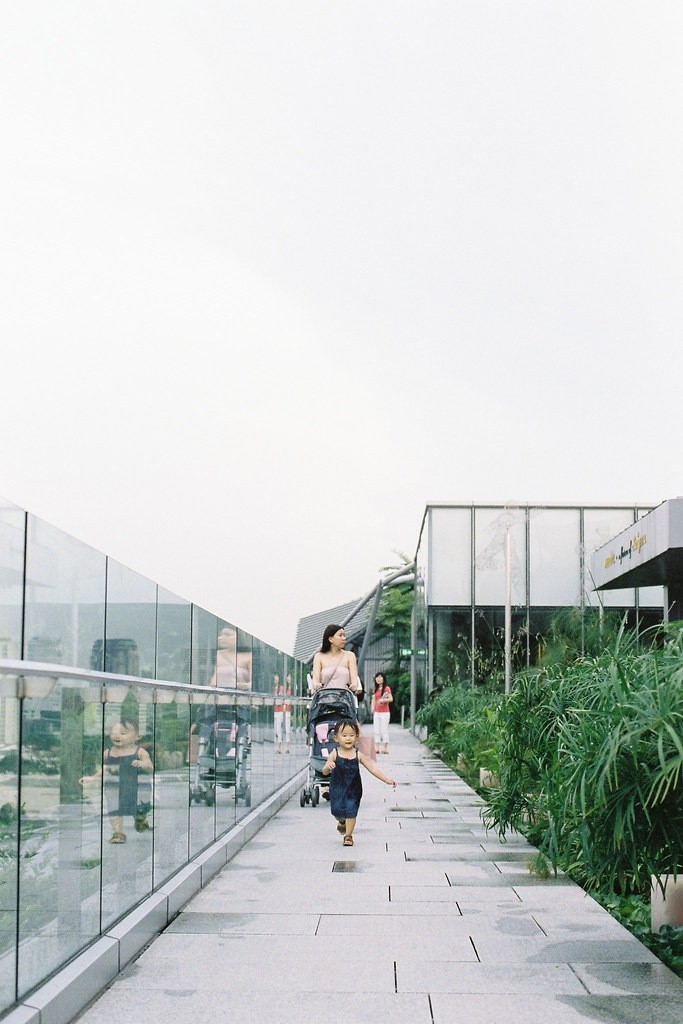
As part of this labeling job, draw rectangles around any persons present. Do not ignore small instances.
[371,671,393,754]
[204,626,254,707]
[352,674,363,715]
[310,624,358,694]
[77,714,154,844]
[273,667,313,755]
[322,719,398,846]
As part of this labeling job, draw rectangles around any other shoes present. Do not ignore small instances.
[275,748,290,754]
[322,786,331,801]
[374,749,389,754]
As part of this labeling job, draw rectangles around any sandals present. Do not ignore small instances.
[134,816,148,833]
[337,822,346,835]
[110,833,126,843]
[343,835,353,846]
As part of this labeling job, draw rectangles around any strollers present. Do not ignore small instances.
[184,683,253,809]
[294,681,360,808]
